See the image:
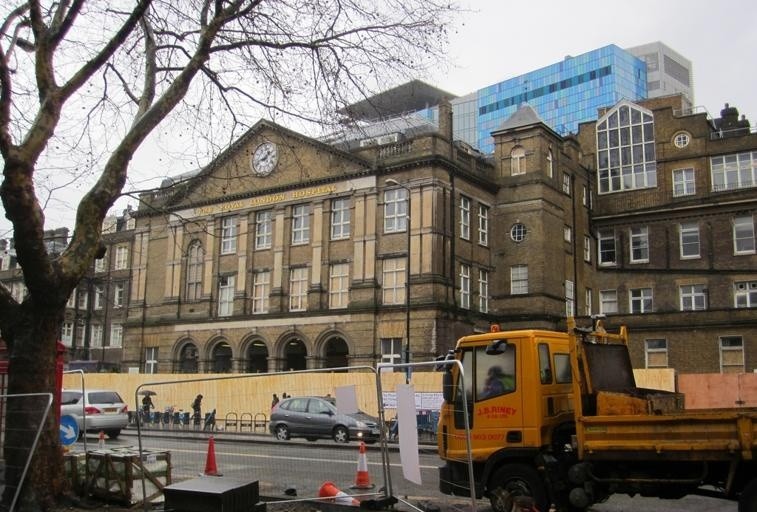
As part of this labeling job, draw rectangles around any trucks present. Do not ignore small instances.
[438,319,757,511]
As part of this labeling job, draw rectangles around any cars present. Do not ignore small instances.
[268,395,380,443]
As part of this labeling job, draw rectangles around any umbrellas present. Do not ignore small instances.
[138,390,157,395]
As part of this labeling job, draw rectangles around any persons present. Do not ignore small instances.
[457,365,515,427]
[271,392,291,408]
[141,393,216,433]
[327,393,331,397]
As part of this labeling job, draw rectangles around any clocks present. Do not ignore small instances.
[250,142,280,177]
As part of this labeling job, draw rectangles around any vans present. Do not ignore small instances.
[63,390,129,440]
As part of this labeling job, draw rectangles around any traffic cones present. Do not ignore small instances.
[320,482,360,505]
[204,436,222,476]
[97,432,104,449]
[350,442,376,491]
